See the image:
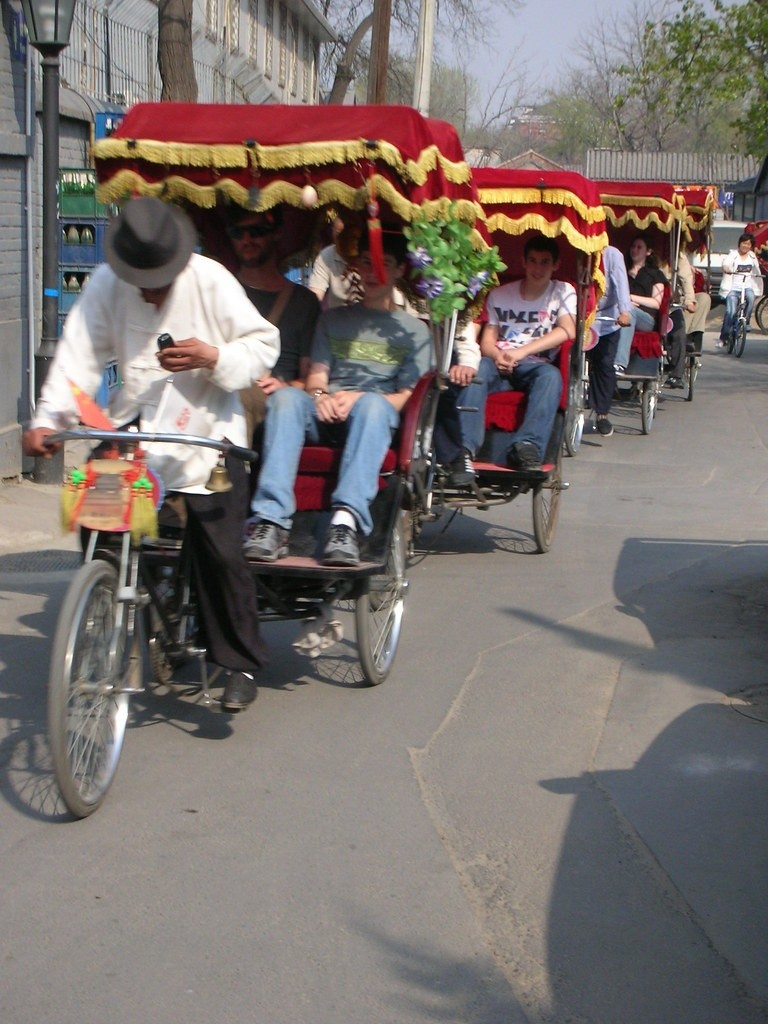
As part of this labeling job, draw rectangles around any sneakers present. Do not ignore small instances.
[614,365,625,375]
[222,670,258,708]
[320,524,361,566]
[597,418,614,437]
[664,377,684,389]
[506,441,542,470]
[447,447,475,488]
[242,521,290,561]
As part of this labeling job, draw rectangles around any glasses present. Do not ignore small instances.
[228,222,272,239]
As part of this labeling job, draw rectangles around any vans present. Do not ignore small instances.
[693,220,747,301]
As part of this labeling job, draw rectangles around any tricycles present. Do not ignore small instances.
[44,101,506,819]
[419,167,609,552]
[659,186,713,401]
[745,221,768,335]
[565,182,687,458]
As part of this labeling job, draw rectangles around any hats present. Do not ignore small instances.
[104,198,198,289]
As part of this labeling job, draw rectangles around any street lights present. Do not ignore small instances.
[18,0,77,483]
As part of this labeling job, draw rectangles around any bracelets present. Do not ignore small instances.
[306,388,328,399]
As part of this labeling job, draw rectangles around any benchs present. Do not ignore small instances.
[238,374,422,511]
[591,284,671,370]
[439,329,577,470]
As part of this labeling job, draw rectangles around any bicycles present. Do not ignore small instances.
[723,271,766,358]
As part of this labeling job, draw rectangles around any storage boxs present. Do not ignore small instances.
[60,187,118,408]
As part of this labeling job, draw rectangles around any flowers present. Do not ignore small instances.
[409,214,500,327]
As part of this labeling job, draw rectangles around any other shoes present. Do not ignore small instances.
[741,325,750,332]
[714,340,724,348]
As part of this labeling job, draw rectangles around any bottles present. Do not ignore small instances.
[54,168,100,294]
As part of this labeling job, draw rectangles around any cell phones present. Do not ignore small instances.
[158,334,175,350]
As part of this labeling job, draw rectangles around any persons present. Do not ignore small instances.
[591,236,631,437]
[715,234,764,348]
[23,198,281,708]
[228,211,437,566]
[613,236,670,375]
[391,235,578,487]
[667,252,712,388]
